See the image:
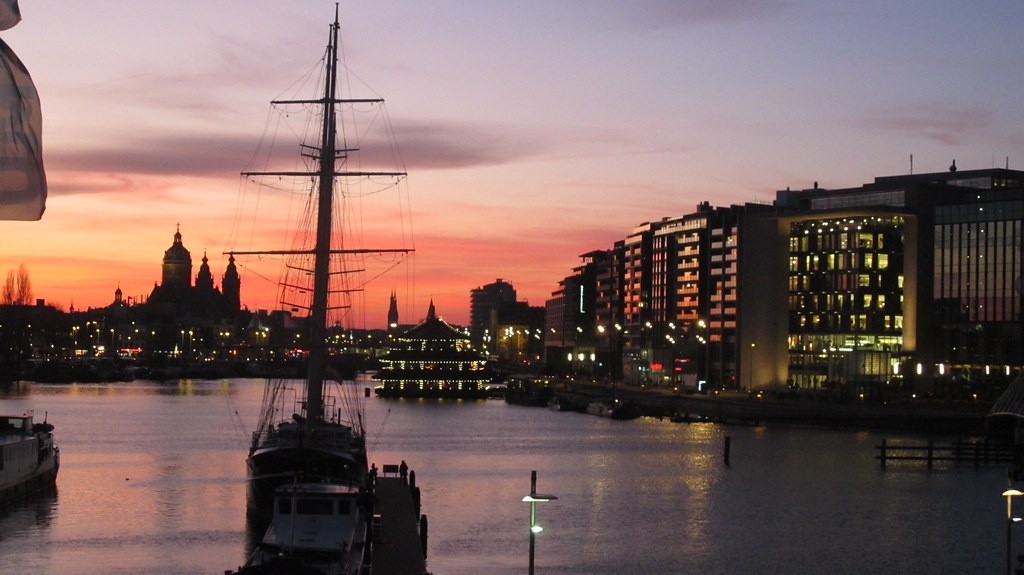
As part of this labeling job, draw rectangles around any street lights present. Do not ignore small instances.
[521,467,556,574]
[915,361,924,395]
[1000,474,1023,574]
[985,364,991,394]
[893,359,900,394]
[934,361,945,397]
[1004,364,1011,389]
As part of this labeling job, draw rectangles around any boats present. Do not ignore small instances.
[0,411,62,513]
[245,407,369,532]
[222,482,377,575]
